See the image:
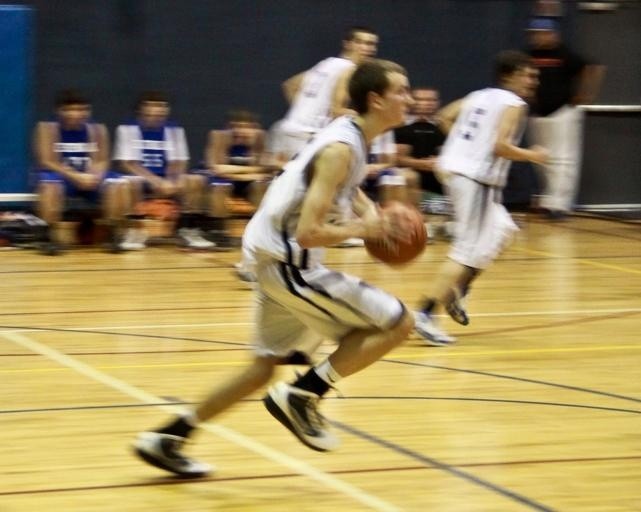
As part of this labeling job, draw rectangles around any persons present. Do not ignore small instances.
[131,60,417,478]
[523,17,607,216]
[394,88,453,245]
[110,92,216,251]
[360,129,407,207]
[202,109,286,242]
[232,27,378,286]
[412,51,547,345]
[32,91,132,250]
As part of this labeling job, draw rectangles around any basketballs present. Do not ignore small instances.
[364,202,425,266]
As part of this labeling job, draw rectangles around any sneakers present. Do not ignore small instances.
[263,381,332,451]
[117,240,145,253]
[180,234,217,249]
[442,288,469,327]
[410,311,456,347]
[136,431,212,476]
